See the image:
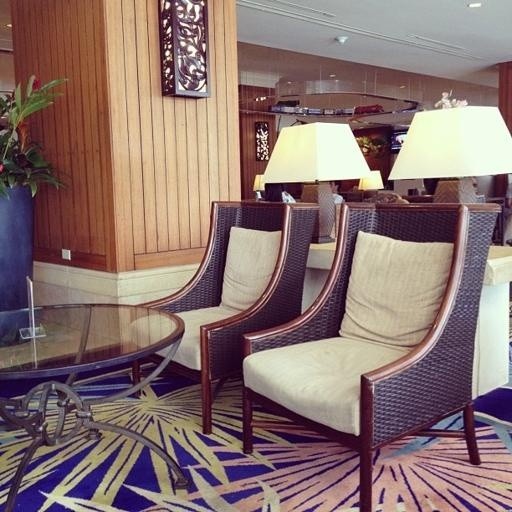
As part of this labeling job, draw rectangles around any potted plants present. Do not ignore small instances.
[0,73,69,348]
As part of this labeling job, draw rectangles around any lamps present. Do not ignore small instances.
[252,105,512,245]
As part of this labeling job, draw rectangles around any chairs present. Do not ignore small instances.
[131,200,321,436]
[240,201,502,511]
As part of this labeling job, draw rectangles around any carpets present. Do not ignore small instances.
[1,343,512,511]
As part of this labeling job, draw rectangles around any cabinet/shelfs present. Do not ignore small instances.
[301,241,512,401]
